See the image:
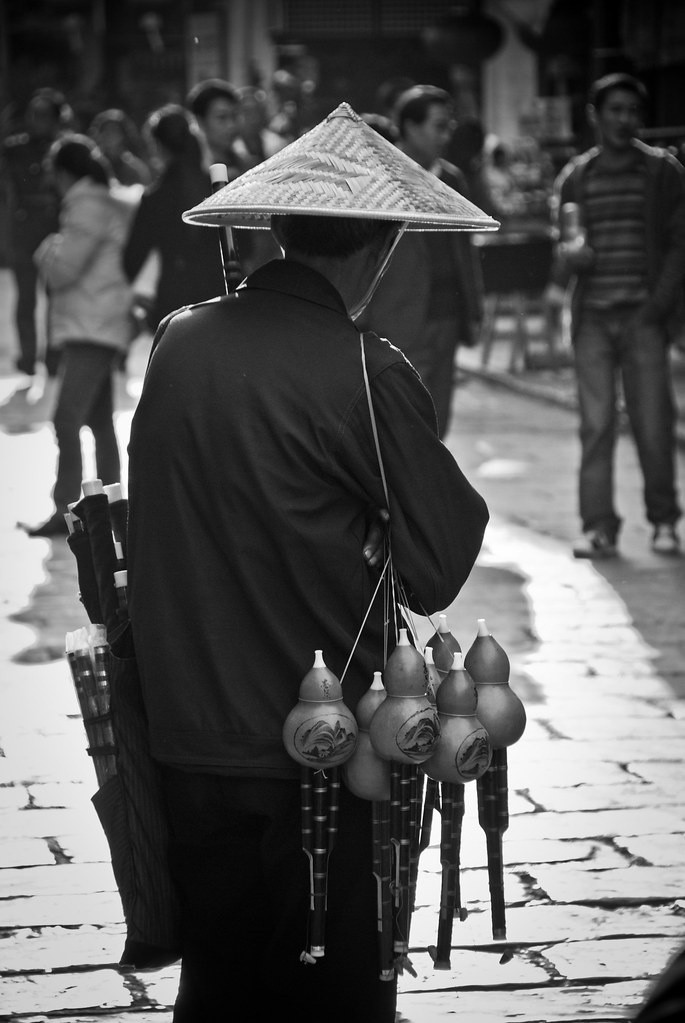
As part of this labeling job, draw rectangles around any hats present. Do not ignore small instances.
[182,100,501,232]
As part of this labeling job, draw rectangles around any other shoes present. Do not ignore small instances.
[30,515,70,535]
[653,520,681,552]
[574,529,615,556]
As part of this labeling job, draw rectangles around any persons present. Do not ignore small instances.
[1,85,80,375]
[341,83,487,441]
[26,132,136,537]
[550,72,685,561]
[126,102,501,1022]
[121,103,250,336]
[88,83,583,283]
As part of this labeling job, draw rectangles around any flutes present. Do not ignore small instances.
[64,645,119,787]
[284,613,524,982]
